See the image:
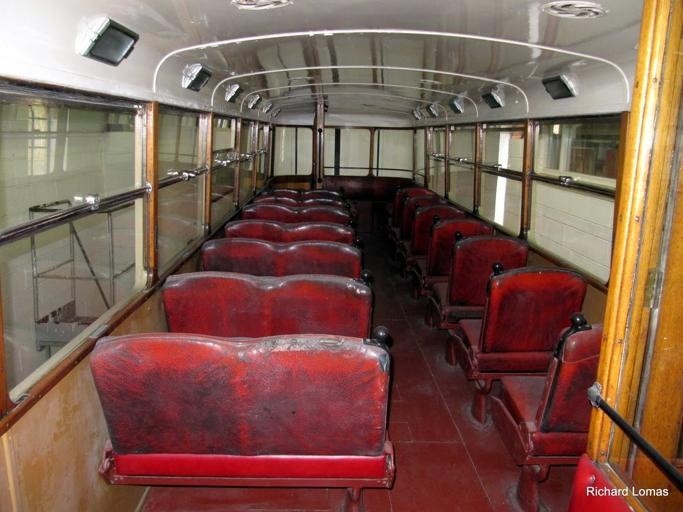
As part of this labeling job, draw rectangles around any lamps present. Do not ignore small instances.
[225,84,246,103]
[249,93,263,109]
[481,92,503,110]
[83,19,140,67]
[182,64,214,92]
[542,75,577,100]
[411,104,438,120]
[449,99,465,114]
[264,104,283,118]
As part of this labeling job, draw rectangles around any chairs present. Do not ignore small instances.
[390,187,433,258]
[255,197,351,206]
[421,213,495,329]
[88,324,397,501]
[451,263,588,424]
[224,220,360,241]
[407,203,465,299]
[262,188,346,201]
[491,313,609,512]
[200,234,370,271]
[243,204,357,224]
[400,192,441,281]
[434,229,529,365]
[163,268,378,337]
